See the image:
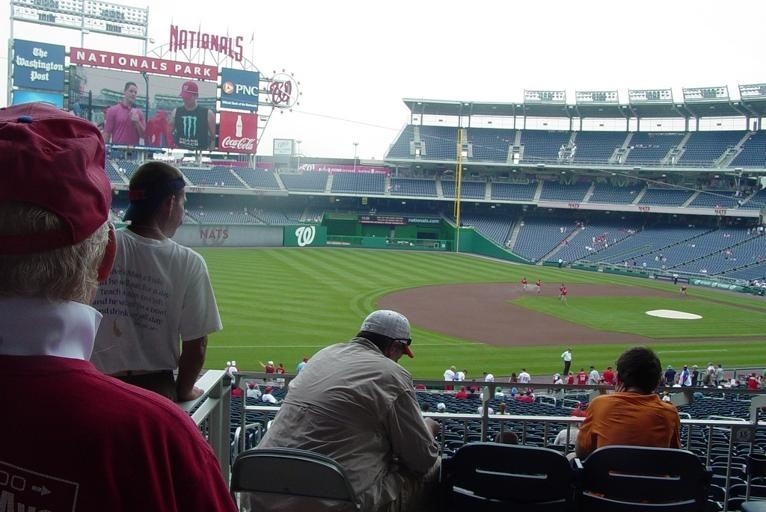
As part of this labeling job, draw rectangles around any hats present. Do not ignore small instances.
[179,81,198,99]
[438,403,446,410]
[1,101,111,252]
[361,309,414,359]
[121,177,185,222]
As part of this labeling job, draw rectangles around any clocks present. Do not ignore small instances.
[262,67,302,115]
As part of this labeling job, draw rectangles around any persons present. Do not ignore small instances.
[0,100,240,512]
[86,160,223,403]
[420,365,535,445]
[224,358,307,405]
[661,361,763,404]
[673,272,679,284]
[165,82,216,150]
[575,346,681,461]
[552,347,617,385]
[554,416,580,446]
[239,310,442,512]
[521,276,569,305]
[680,285,688,296]
[102,81,147,159]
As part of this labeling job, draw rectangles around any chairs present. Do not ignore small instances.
[102,155,390,245]
[382,124,540,253]
[190,371,765,512]
[523,124,765,285]
